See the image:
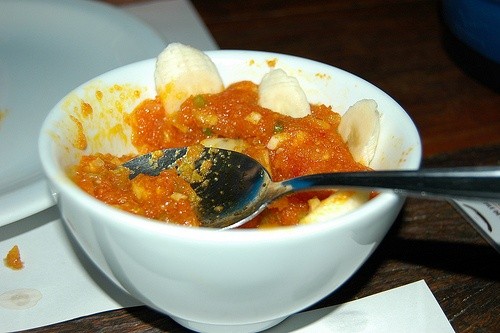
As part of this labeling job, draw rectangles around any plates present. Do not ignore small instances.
[1,1,219,228]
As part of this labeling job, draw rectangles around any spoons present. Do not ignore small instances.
[121,149,500,231]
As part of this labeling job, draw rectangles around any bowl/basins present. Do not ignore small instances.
[39,48,421,333]
